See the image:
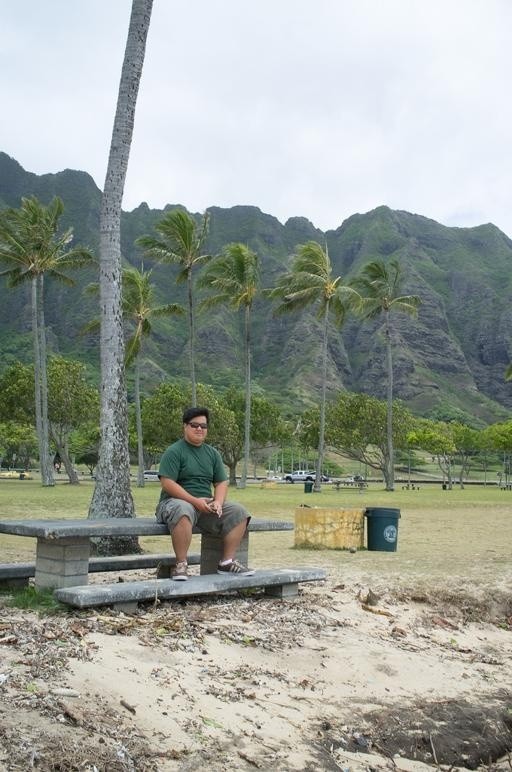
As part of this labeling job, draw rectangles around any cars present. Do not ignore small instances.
[143,469,160,479]
[302,471,329,482]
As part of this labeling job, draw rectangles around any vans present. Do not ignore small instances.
[283,471,311,484]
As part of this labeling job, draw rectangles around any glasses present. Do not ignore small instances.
[187,422,207,428]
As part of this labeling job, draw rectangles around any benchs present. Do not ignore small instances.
[0,517,328,610]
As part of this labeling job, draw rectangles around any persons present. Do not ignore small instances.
[154,406,257,584]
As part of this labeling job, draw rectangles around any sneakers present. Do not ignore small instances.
[217,560,254,576]
[172,559,188,581]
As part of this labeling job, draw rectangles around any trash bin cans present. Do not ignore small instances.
[365,507,401,552]
[20,474,25,480]
[305,483,313,493]
